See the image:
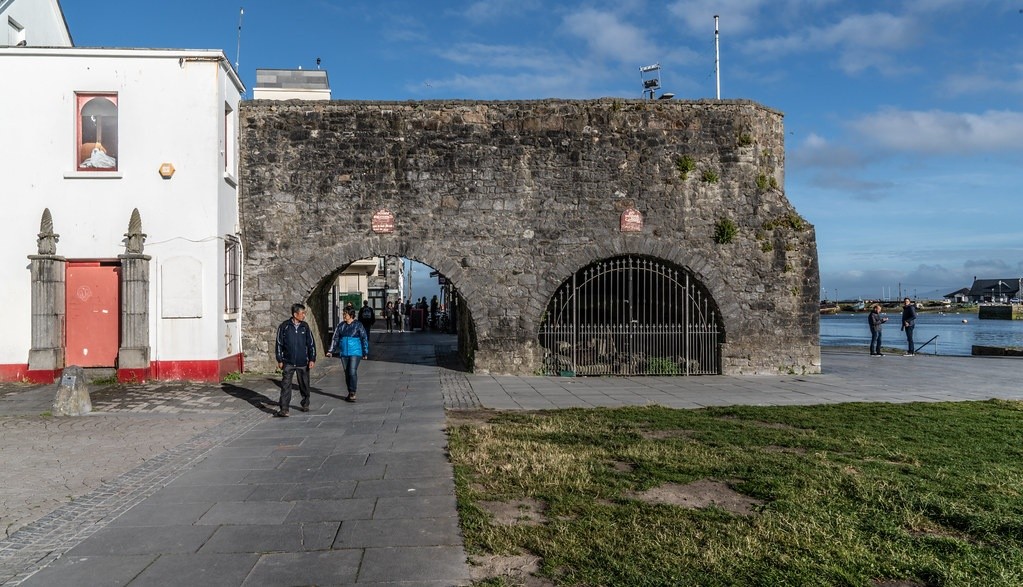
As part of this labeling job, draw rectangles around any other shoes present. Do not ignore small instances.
[398,330,400,333]
[401,329,405,332]
[303,404,309,412]
[876,352,886,357]
[387,330,390,334]
[277,409,289,417]
[391,330,393,334]
[349,391,356,402]
[903,352,914,357]
[344,393,350,402]
[869,353,881,357]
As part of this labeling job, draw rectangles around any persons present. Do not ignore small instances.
[344,295,438,342]
[899,299,918,356]
[273,303,317,417]
[326,306,368,401]
[867,304,890,358]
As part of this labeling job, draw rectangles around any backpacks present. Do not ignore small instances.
[362,306,371,319]
